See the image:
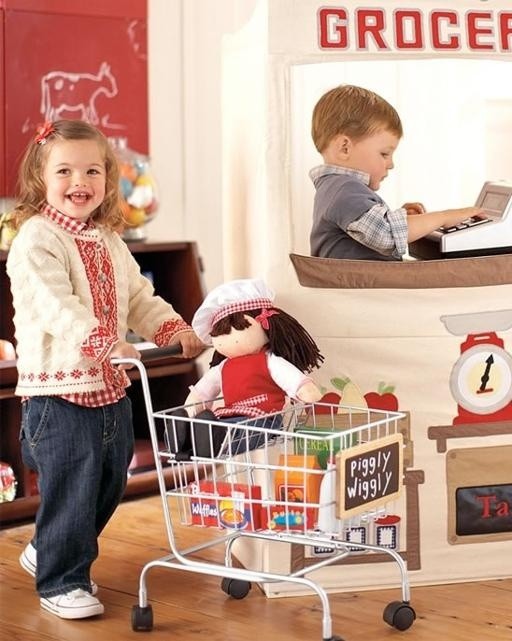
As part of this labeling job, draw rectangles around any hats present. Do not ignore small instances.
[191,277,276,347]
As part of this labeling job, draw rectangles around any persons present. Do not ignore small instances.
[4,116,204,625]
[164,274,324,461]
[303,84,489,261]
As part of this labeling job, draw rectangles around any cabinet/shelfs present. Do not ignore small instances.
[0,240,223,524]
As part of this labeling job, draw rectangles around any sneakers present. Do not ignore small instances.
[16,540,102,599]
[36,583,107,621]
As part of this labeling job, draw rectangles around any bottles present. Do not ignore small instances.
[106,134,158,241]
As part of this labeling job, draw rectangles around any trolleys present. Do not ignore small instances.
[106,337,423,641]
